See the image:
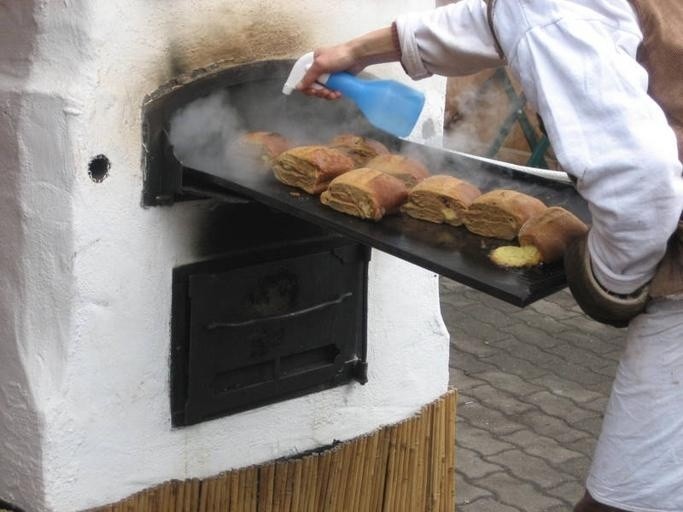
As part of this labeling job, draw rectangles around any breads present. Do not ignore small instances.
[271,146,356,195]
[519,208,589,267]
[363,154,430,190]
[320,168,411,221]
[400,175,483,227]
[458,189,549,240]
[223,129,300,178]
[326,134,390,169]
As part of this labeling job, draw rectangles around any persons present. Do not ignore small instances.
[294,1,682,512]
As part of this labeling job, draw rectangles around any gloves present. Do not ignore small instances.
[563,227,651,327]
